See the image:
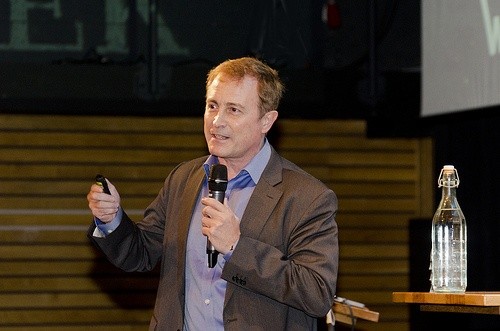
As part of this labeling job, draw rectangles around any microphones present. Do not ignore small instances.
[205,164,228,268]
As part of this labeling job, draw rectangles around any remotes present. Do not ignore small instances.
[96,175,112,196]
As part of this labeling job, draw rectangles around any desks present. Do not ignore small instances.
[394,291,500,314]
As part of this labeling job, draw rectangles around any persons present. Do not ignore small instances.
[85,57,338,331]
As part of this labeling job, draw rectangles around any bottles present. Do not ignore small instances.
[431,165,468,292]
